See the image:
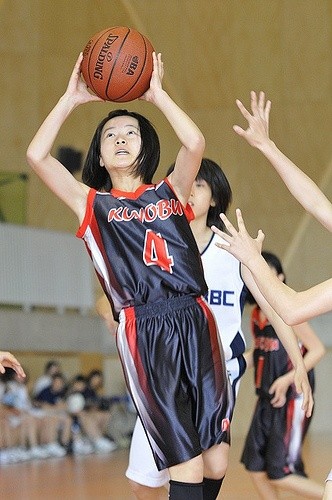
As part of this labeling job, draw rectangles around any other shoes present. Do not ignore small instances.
[0,436,118,465]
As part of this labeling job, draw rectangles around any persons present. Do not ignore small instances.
[211,89,332,325]
[0,352,119,464]
[25,26,232,500]
[126,159,314,500]
[240,252,332,500]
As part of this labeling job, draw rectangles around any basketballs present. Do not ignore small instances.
[80,25,156,104]
